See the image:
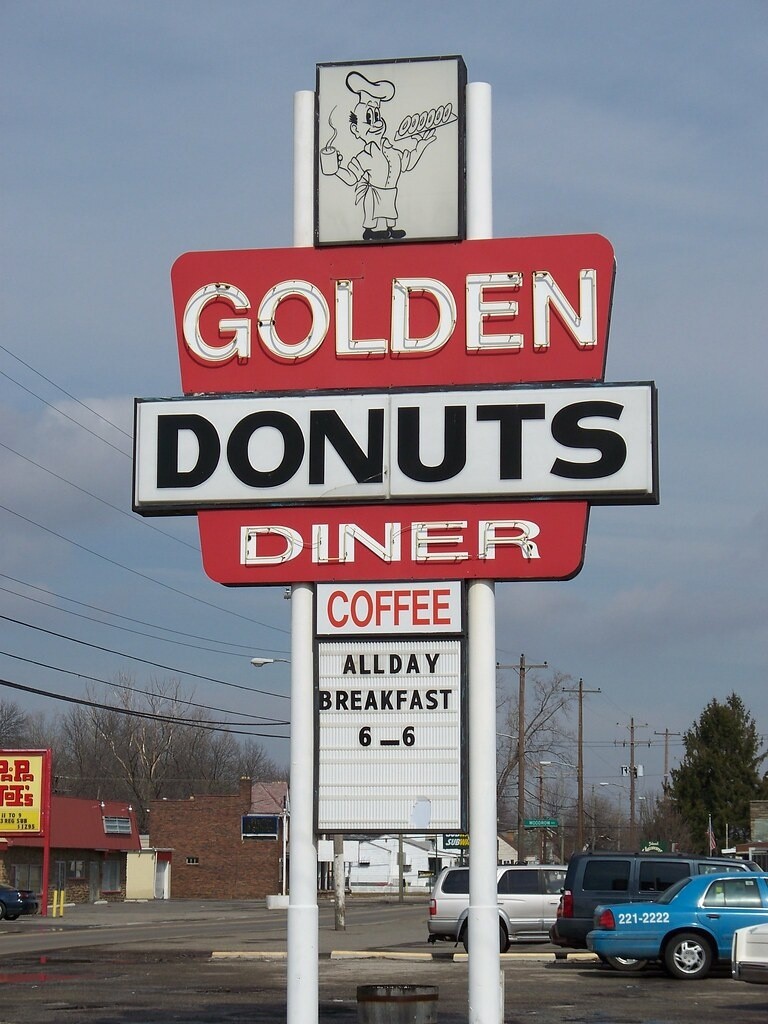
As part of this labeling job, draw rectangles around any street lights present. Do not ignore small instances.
[638,796,671,851]
[599,782,636,849]
[539,761,584,851]
[249,657,348,931]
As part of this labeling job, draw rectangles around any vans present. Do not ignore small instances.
[426,865,568,954]
[547,851,768,973]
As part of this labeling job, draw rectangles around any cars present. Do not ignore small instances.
[582,871,768,981]
[0,882,39,921]
[731,922,768,983]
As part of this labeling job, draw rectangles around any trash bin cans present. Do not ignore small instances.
[356,981,438,1024]
